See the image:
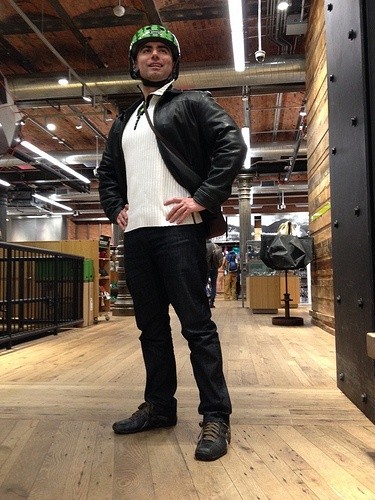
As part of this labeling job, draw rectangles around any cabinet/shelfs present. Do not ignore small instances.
[0,240,111,328]
[245,274,299,314]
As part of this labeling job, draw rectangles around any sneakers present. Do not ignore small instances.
[112,397,177,435]
[195,412,230,461]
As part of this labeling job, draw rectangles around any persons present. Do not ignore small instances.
[98,26,248,462]
[204,239,255,309]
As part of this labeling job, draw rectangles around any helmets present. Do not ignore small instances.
[129,25,181,82]
